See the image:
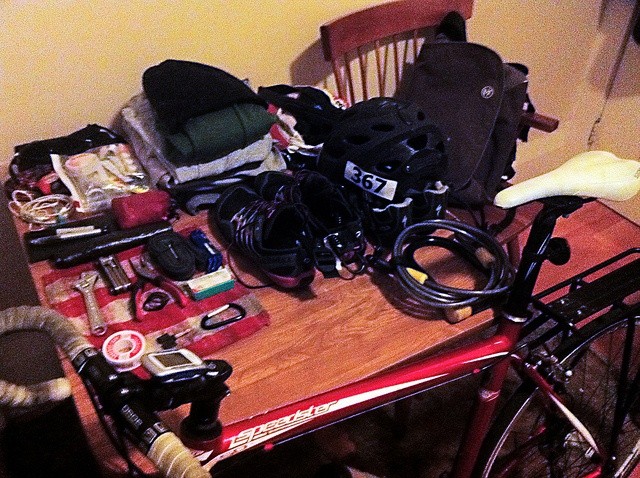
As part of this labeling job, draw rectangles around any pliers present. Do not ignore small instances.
[127,253,188,321]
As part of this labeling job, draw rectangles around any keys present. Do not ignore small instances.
[157,328,191,349]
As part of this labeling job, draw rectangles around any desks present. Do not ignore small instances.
[8,200,514,473]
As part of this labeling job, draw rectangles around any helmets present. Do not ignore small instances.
[316,96,453,249]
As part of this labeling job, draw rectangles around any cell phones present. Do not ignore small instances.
[141,347,203,376]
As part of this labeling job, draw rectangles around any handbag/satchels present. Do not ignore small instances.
[392,32,528,207]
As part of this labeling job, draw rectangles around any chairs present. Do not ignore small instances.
[321,1,559,269]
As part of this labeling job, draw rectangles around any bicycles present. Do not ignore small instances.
[0,149,640,478]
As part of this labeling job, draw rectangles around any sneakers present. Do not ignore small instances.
[253,171,368,264]
[212,182,337,289]
